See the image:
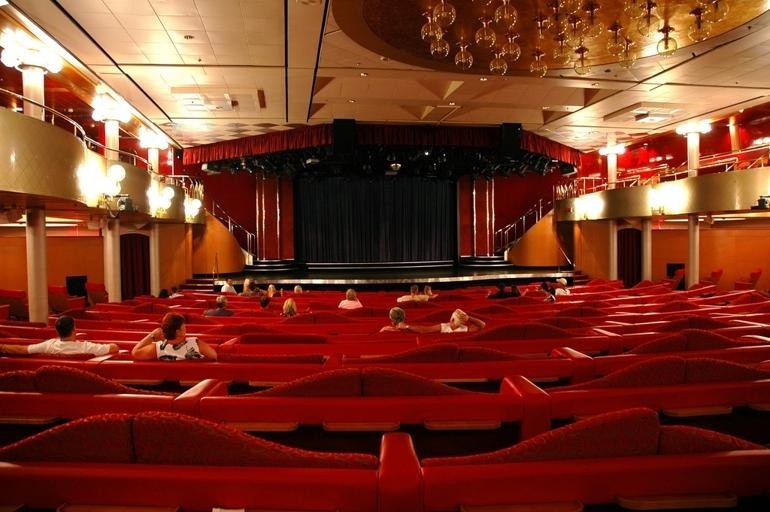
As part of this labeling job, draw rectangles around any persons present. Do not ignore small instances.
[159,288,184,299]
[128,313,220,361]
[489,277,570,302]
[338,289,362,309]
[220,278,281,297]
[260,285,302,317]
[2,314,120,358]
[213,295,227,311]
[379,307,486,334]
[397,285,437,302]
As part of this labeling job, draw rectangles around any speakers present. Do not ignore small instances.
[66,276,88,306]
[331,119,356,158]
[667,263,685,292]
[498,123,522,157]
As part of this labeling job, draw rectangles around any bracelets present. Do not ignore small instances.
[150,331,158,342]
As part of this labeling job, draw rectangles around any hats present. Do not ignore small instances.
[556,278,567,285]
